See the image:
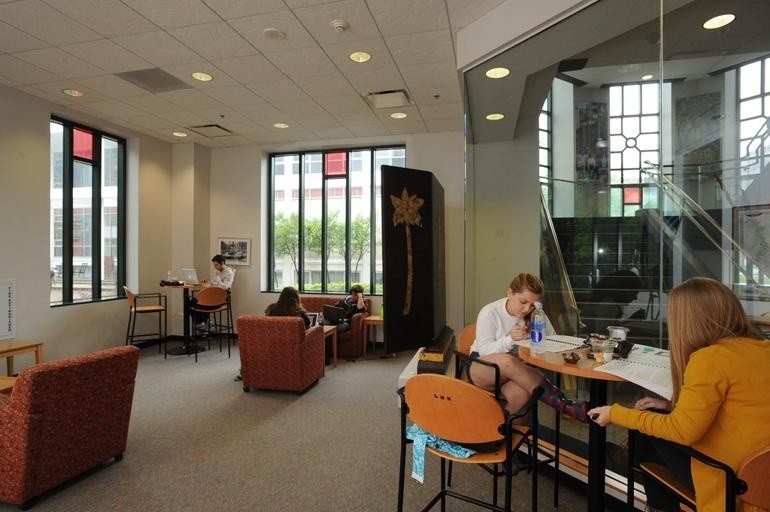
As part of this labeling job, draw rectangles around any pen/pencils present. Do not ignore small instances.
[592,414,598,419]
[516,323,528,331]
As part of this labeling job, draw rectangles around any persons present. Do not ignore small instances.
[588,275,769,512]
[593,269,646,345]
[185,252,236,330]
[263,286,310,330]
[466,270,590,479]
[322,283,369,366]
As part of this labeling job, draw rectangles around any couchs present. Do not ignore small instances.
[301,296,372,359]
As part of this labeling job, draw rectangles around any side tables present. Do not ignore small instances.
[361,312,384,357]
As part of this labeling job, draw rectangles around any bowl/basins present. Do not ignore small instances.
[563,351,581,364]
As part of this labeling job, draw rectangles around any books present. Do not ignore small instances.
[513,334,588,354]
[592,341,675,402]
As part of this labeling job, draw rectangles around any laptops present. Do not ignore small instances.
[181,269,208,285]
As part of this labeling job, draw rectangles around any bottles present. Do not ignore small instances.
[380,304,383,319]
[529,301,545,357]
[318,310,324,326]
[168,269,172,281]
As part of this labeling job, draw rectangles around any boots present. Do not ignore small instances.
[502,417,528,476]
[539,377,590,423]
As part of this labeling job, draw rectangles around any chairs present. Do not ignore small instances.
[393,326,541,509]
[237,315,326,394]
[122,286,170,361]
[1,345,141,509]
[189,288,232,360]
[640,446,770,510]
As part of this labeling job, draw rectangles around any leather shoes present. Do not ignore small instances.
[606,442,628,467]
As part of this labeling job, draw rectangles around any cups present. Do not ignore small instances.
[592,323,630,364]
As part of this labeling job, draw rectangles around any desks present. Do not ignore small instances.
[162,282,211,355]
[518,335,659,509]
[0,337,47,396]
